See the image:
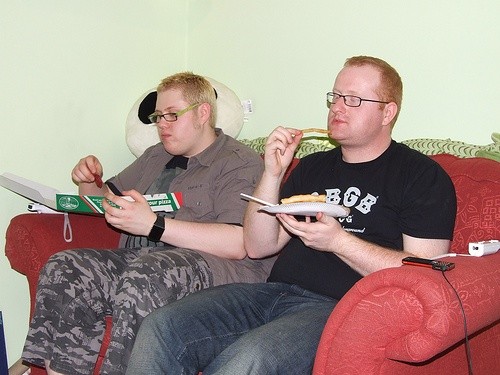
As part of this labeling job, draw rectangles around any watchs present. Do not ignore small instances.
[148,216,165,241]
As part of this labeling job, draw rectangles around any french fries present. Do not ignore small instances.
[299,128,333,134]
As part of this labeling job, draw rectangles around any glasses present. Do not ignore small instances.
[148,103,201,122]
[327,92,389,107]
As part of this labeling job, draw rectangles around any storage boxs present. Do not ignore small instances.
[0,173,183,214]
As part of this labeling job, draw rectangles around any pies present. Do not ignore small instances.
[281,194,326,204]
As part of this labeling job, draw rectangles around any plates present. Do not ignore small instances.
[257,203,348,217]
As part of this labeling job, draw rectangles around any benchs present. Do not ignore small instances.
[5,138,500,375]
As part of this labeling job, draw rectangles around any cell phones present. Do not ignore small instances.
[403,257,455,272]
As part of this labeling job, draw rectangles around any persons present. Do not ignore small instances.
[124,56,457,375]
[20,71,280,375]
